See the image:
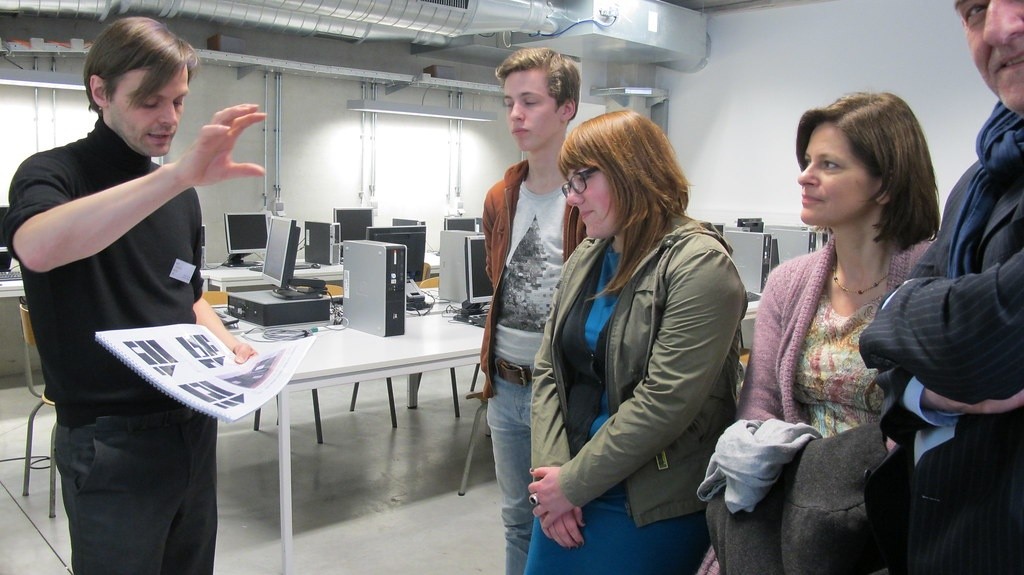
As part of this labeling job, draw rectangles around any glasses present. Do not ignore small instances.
[561,165,599,199]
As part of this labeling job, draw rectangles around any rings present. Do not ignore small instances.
[528,494,538,506]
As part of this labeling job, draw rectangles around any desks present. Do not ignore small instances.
[211,288,486,575]
[209,256,442,292]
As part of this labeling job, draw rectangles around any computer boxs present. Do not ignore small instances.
[724,226,831,292]
[227,217,485,338]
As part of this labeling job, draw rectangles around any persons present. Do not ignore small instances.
[525,110,747,575]
[696,91,939,575]
[859,0,1024,575]
[9,17,266,575]
[480,49,589,575]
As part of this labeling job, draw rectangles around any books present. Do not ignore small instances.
[93,323,317,423]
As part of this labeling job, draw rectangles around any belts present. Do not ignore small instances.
[489,357,535,384]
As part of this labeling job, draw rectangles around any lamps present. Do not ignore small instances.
[347,99,498,122]
[0,66,88,91]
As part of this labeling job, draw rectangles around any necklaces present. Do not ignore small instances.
[832,260,889,294]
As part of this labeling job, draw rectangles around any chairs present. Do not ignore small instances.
[18,262,491,520]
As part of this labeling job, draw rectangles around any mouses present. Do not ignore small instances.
[311,263,321,269]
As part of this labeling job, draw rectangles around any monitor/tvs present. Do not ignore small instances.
[366,225,429,311]
[770,239,780,271]
[453,235,493,322]
[222,213,267,268]
[736,218,764,234]
[0,204,13,272]
[444,218,476,233]
[262,216,323,300]
[713,223,725,237]
[333,207,374,258]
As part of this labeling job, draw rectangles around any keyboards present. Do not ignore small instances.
[469,313,488,328]
[214,310,239,326]
[0,271,22,281]
[248,264,310,272]
